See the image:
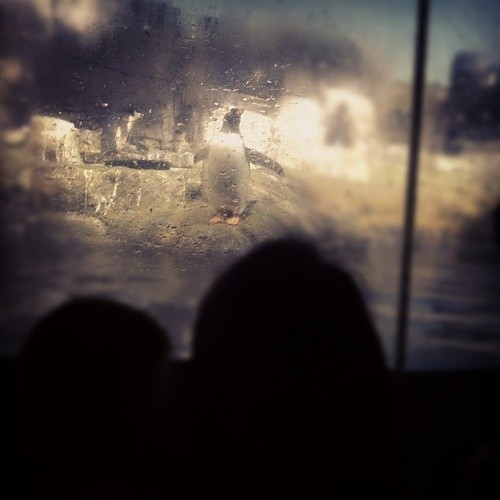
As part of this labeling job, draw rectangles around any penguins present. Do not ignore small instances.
[193,107,285,227]
[102,108,144,152]
